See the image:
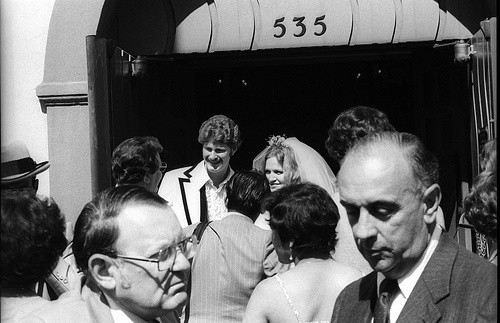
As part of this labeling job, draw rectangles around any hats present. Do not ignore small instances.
[1,139,50,184]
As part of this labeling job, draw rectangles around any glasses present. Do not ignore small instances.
[159,162,168,172]
[109,234,198,271]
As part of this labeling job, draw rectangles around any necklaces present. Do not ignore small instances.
[297,259,326,264]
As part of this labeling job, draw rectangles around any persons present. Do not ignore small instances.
[1,189,67,323]
[112,136,167,193]
[158,115,243,229]
[325,106,398,166]
[241,182,365,323]
[329,132,497,323]
[18,185,199,323]
[183,168,294,323]
[1,140,84,301]
[253,136,374,273]
[464,138,497,266]
